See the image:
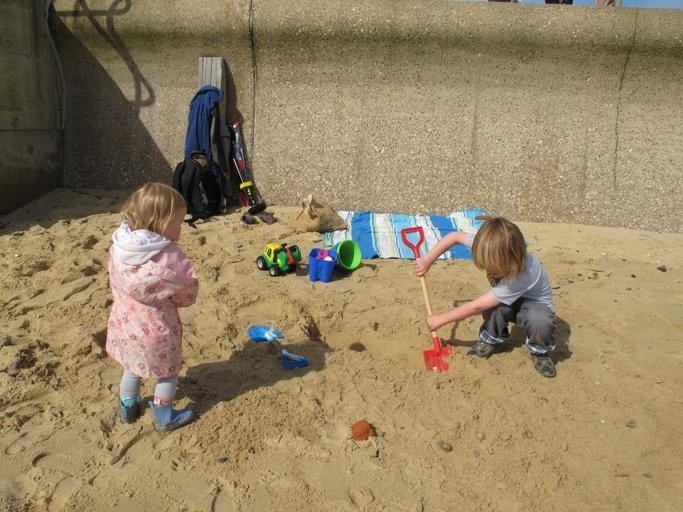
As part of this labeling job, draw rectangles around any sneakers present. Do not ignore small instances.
[529,350,557,378]
[466,338,509,359]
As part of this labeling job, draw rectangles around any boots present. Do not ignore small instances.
[147,400,194,433]
[119,392,142,425]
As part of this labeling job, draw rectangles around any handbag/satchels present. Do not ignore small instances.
[293,193,348,233]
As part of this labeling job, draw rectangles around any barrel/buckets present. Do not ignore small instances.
[335,239,363,270]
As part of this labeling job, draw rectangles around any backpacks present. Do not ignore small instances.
[171,149,229,229]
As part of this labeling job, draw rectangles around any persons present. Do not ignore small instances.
[104,182,199,433]
[413,216,559,377]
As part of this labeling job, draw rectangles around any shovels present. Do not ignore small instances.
[246,324,310,369]
[401,226,454,374]
